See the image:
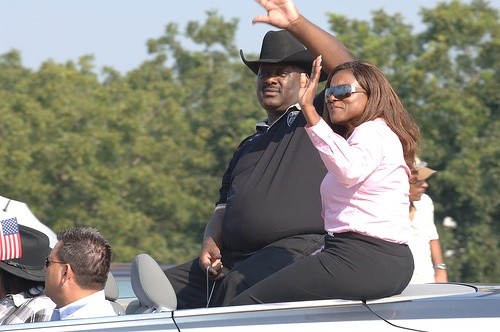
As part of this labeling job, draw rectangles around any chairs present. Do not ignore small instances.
[121,252,177,317]
[101,268,125,316]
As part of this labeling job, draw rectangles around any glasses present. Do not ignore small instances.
[45,256,66,267]
[325,84,367,104]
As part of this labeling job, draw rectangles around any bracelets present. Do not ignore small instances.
[434,263,446,270]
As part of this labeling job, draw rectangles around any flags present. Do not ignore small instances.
[0,217,22,261]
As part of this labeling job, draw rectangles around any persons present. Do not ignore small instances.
[407,156,447,284]
[164,0,357,309]
[0,224,58,325]
[224,55,419,306]
[44,226,118,321]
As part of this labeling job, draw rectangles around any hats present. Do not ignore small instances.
[414,158,437,181]
[240,29,328,83]
[0,224,52,281]
